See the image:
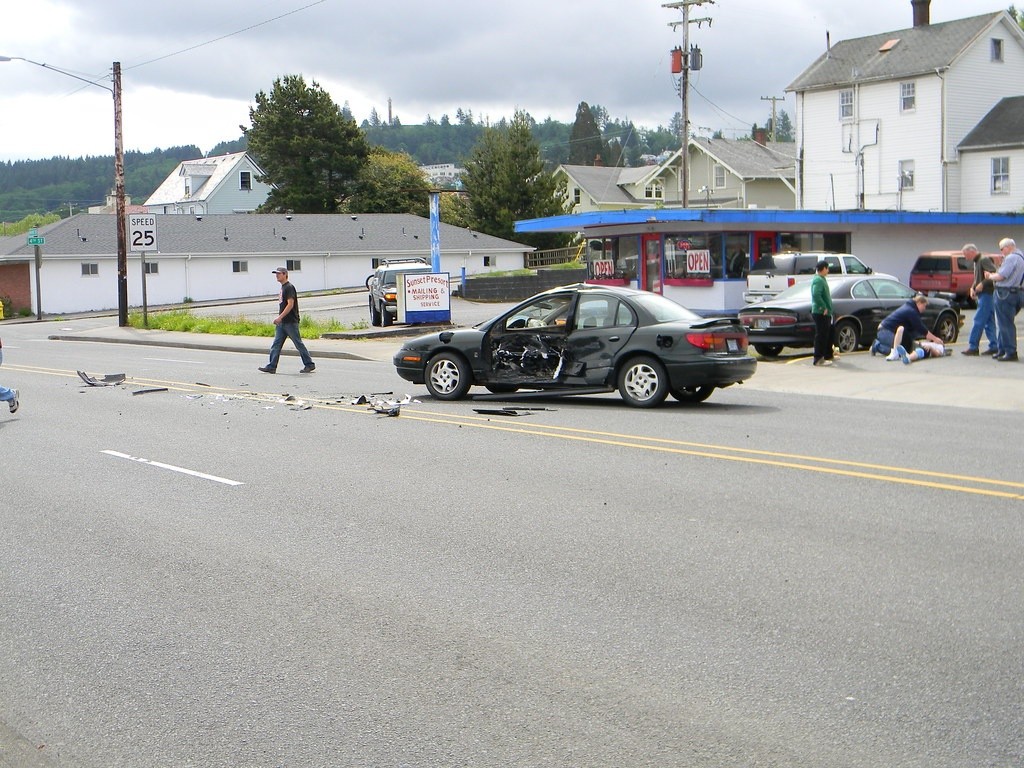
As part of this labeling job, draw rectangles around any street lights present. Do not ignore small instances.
[0,55,128,327]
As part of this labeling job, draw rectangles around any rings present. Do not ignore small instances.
[277,323,278,325]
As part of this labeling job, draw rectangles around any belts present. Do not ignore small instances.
[998,287,1022,290]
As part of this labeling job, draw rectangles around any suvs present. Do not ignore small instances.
[743,250,899,304]
[369,257,432,328]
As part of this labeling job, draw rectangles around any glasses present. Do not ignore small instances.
[1001,247,1005,251]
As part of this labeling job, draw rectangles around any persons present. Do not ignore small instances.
[961,244,999,356]
[869,296,945,365]
[0,337,19,413]
[811,260,840,366]
[729,240,745,278]
[258,267,315,374]
[984,238,1024,362]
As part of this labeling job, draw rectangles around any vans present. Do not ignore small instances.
[909,251,1005,310]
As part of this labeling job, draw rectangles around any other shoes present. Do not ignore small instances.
[981,349,997,355]
[8,389,20,413]
[961,349,979,356]
[815,360,833,365]
[886,348,899,361]
[257,364,276,374]
[998,351,1018,361]
[870,339,880,356]
[832,356,840,360]
[897,345,910,364]
[300,365,315,373]
[992,349,1005,358]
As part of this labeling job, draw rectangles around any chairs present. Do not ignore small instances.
[879,285,897,297]
[583,317,597,328]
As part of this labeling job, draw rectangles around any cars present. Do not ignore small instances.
[392,281,757,409]
[736,277,965,357]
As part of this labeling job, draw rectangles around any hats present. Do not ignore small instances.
[272,267,287,274]
